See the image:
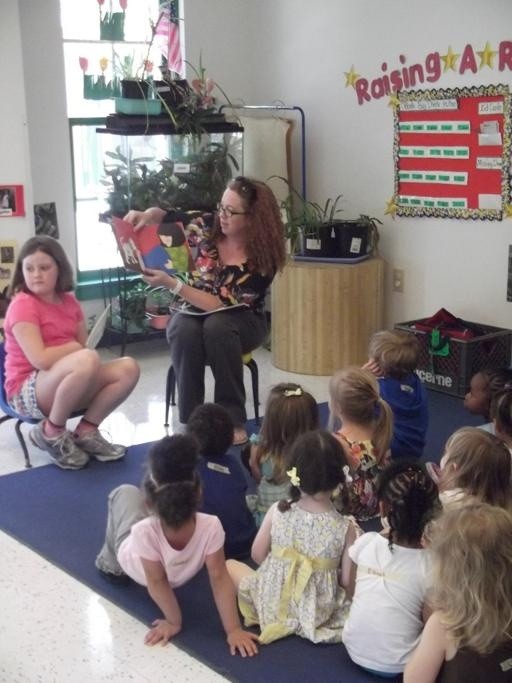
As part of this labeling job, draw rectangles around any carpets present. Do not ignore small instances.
[0,388,485,683]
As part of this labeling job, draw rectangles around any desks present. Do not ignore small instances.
[270,257,384,376]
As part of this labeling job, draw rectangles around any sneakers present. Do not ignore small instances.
[69,423,128,462]
[29,418,89,472]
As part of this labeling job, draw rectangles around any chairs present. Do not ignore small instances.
[0,340,41,467]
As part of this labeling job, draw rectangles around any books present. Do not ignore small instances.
[108,214,196,276]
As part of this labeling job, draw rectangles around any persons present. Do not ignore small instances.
[122,178,287,447]
[94,328,511,682]
[2,237,140,469]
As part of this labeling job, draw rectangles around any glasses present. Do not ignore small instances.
[216,201,251,217]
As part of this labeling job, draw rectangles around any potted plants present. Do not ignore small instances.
[111,289,145,335]
[265,175,382,257]
[114,53,148,98]
[141,272,189,330]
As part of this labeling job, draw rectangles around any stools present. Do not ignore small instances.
[165,353,259,427]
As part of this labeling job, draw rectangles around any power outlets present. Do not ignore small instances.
[393,268,403,292]
